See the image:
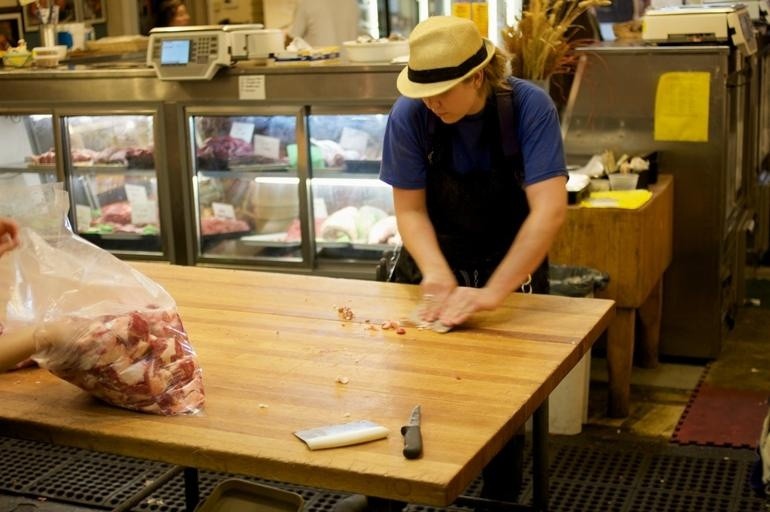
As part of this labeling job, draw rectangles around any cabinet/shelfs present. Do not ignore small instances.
[1,98,406,281]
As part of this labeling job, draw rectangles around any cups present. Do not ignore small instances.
[610,173,639,191]
[33,22,95,67]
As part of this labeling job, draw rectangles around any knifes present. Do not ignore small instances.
[401,404,422,459]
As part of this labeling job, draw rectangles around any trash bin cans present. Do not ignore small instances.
[525,266,610,436]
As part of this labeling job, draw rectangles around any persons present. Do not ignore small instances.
[327,16,570,512]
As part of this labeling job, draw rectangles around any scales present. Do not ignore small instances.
[642,3,758,57]
[146,24,285,80]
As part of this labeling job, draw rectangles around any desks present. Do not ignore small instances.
[0,258,618,512]
[548,173,673,417]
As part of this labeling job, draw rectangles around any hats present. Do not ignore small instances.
[396,16,495,99]
